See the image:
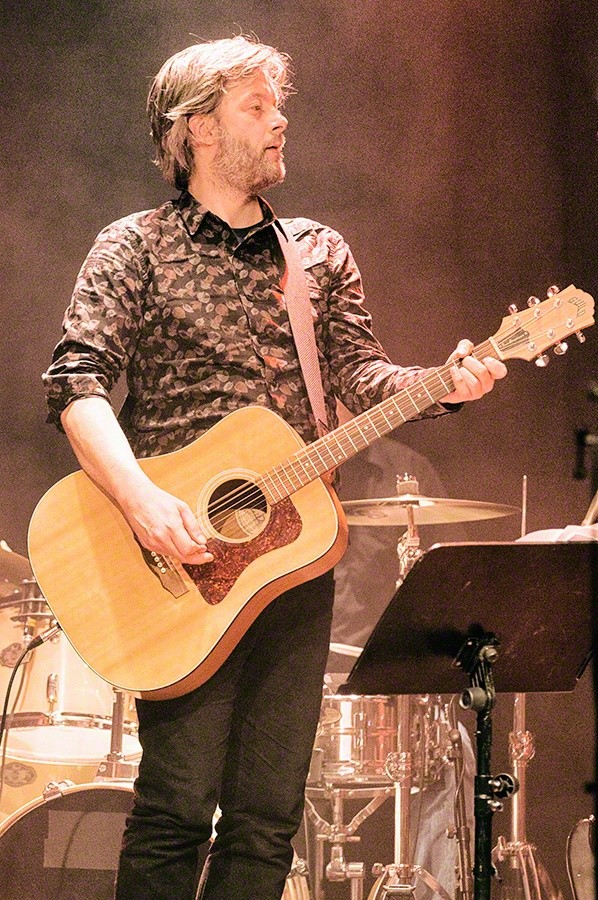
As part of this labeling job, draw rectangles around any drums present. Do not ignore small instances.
[0,776,310,900]
[0,589,144,766]
[304,673,424,794]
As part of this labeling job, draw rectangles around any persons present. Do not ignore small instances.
[42,37,506,900]
[324,396,476,900]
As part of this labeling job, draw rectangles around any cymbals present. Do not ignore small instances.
[339,494,522,526]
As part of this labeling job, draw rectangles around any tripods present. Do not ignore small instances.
[368,694,451,900]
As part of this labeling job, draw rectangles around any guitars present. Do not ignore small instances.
[27,279,598,702]
